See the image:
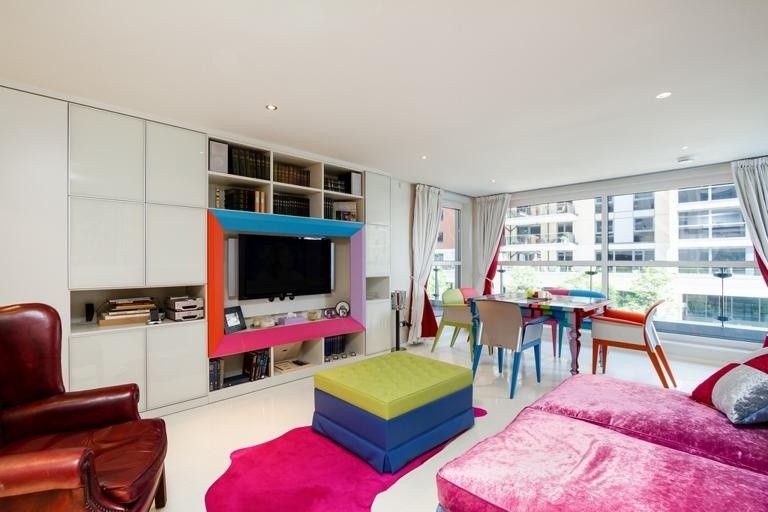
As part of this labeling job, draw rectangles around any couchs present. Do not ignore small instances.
[310,349,474,477]
[527,372,767,478]
[0,303,168,512]
[435,406,768,511]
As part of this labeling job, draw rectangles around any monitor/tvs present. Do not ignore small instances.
[238,233,331,302]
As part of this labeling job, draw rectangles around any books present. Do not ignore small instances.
[225,190,265,212]
[326,177,348,192]
[209,359,224,392]
[323,196,336,218]
[208,139,270,181]
[274,195,310,217]
[325,335,346,356]
[243,350,268,381]
[224,374,250,388]
[274,162,310,186]
[96,298,157,326]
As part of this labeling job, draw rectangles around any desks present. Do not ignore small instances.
[465,292,615,376]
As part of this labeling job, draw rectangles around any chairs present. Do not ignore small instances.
[522,287,569,357]
[431,287,494,362]
[590,298,677,389]
[558,288,606,365]
[459,286,481,304]
[471,299,552,401]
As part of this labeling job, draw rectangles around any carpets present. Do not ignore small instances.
[204,406,488,512]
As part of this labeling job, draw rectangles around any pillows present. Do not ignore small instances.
[687,345,768,425]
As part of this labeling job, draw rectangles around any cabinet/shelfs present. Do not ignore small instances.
[68,95,208,335]
[364,170,392,357]
[68,317,209,422]
[0,79,68,393]
[208,133,367,223]
[209,330,366,406]
